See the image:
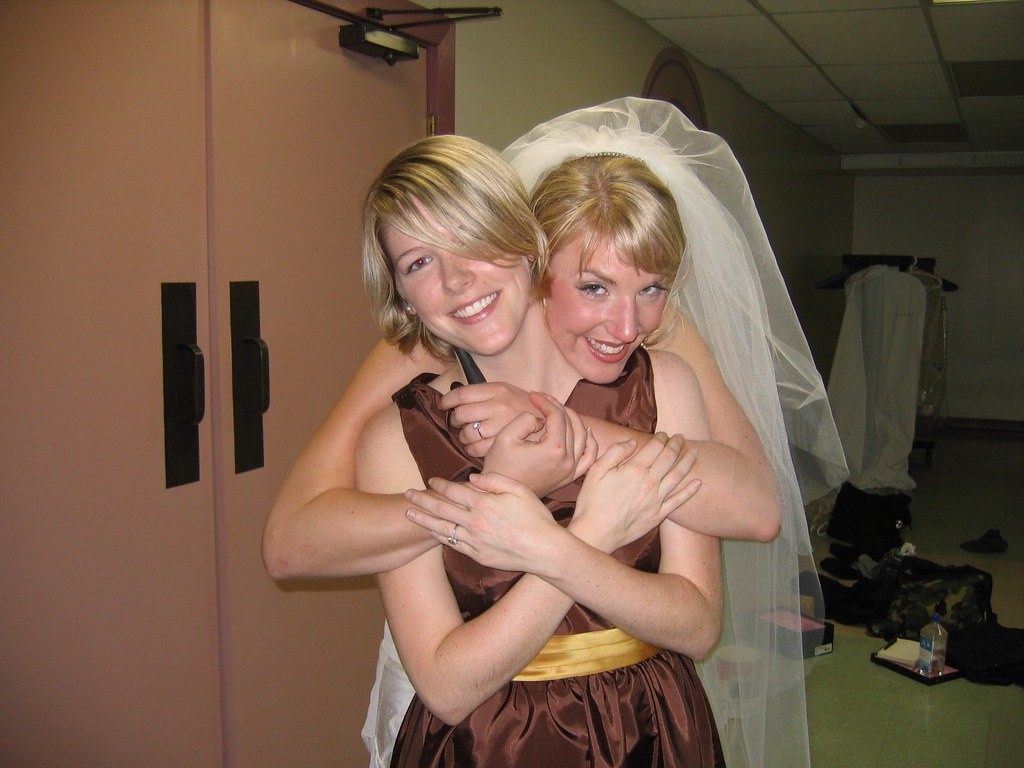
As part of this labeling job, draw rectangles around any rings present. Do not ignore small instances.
[473,421,485,439]
[447,524,460,545]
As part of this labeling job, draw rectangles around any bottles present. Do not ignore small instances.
[918,613,948,677]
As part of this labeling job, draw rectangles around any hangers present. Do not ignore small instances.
[814,253,960,298]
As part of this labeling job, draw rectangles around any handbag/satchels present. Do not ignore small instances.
[826,480,913,549]
[881,577,984,641]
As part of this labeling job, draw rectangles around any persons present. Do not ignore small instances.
[358,135,728,768]
[264,151,780,768]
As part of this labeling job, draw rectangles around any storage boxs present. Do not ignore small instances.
[748,606,834,661]
